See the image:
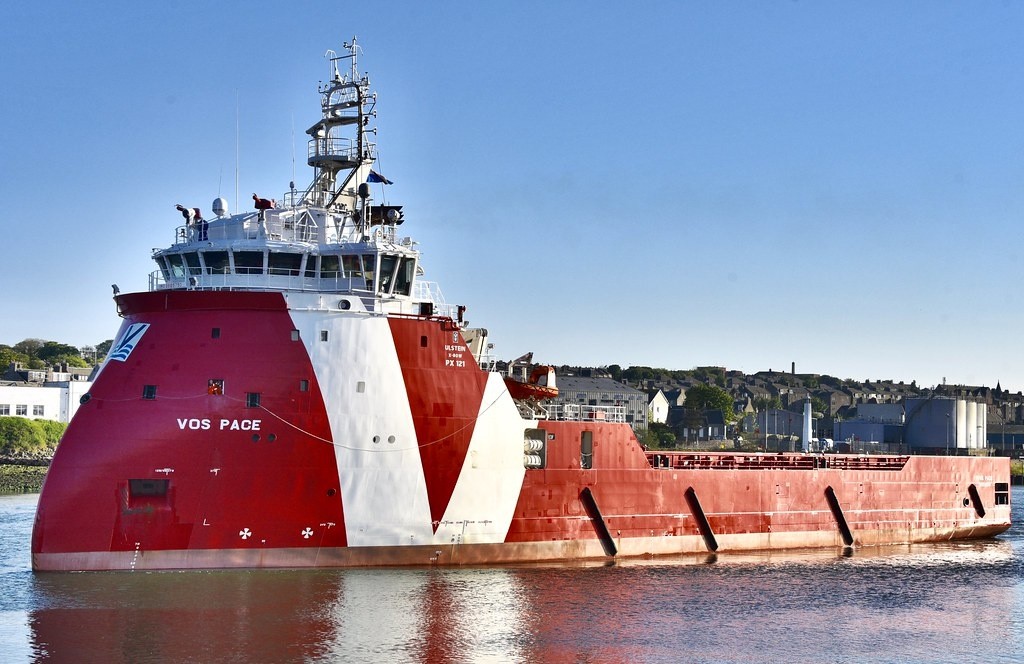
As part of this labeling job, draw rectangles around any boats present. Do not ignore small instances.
[31,35,1011,571]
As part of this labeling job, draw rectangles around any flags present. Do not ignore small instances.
[366,169,394,185]
